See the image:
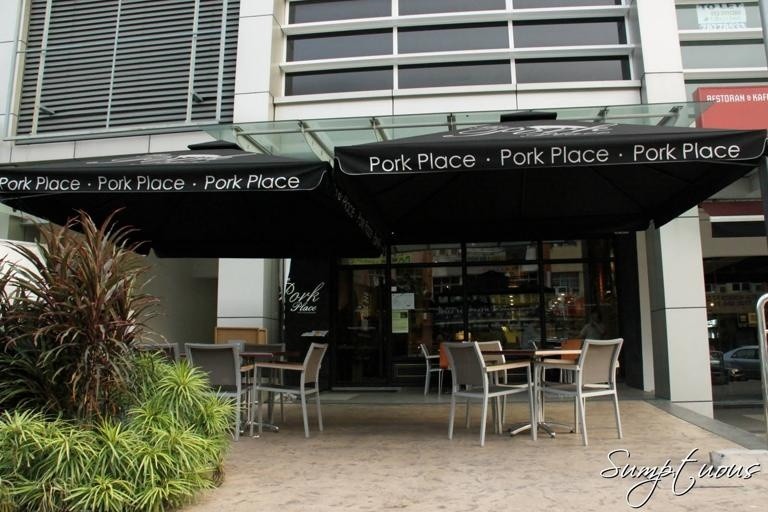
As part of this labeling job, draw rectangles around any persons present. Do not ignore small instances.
[576,307,613,340]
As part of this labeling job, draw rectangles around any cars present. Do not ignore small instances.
[709,344,760,384]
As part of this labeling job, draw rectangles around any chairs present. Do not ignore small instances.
[420,337,626,448]
[141,326,330,441]
